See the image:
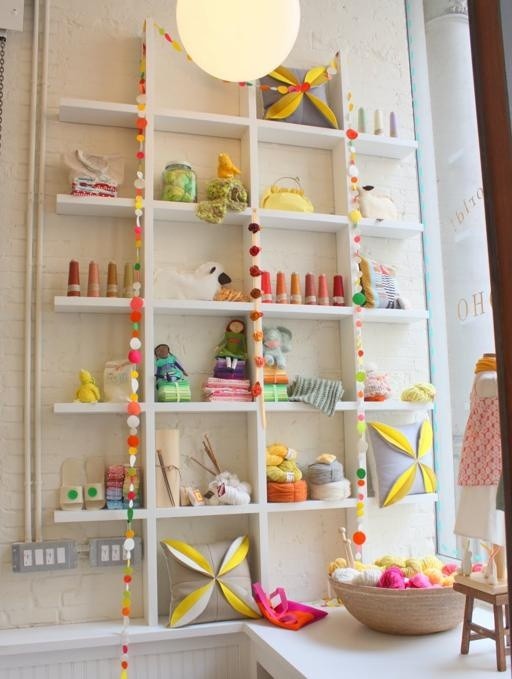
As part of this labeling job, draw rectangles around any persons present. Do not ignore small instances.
[454,353,500,542]
[155,345,190,382]
[213,319,247,373]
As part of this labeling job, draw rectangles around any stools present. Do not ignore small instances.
[453,583,512,672]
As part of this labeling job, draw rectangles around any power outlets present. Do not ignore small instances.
[89,538,143,566]
[12,539,78,573]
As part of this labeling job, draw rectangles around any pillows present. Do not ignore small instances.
[366,414,437,509]
[159,534,263,629]
[259,66,339,130]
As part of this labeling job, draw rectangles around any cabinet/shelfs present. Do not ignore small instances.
[0,631,257,679]
[50,20,438,628]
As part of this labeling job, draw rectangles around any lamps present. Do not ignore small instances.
[176,0,300,82]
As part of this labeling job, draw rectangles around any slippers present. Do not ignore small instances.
[84,459,106,510]
[60,458,84,510]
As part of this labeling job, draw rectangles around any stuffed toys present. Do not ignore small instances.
[262,327,292,370]
[72,369,102,404]
[357,184,397,220]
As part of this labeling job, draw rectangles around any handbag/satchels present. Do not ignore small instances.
[261,177,314,212]
